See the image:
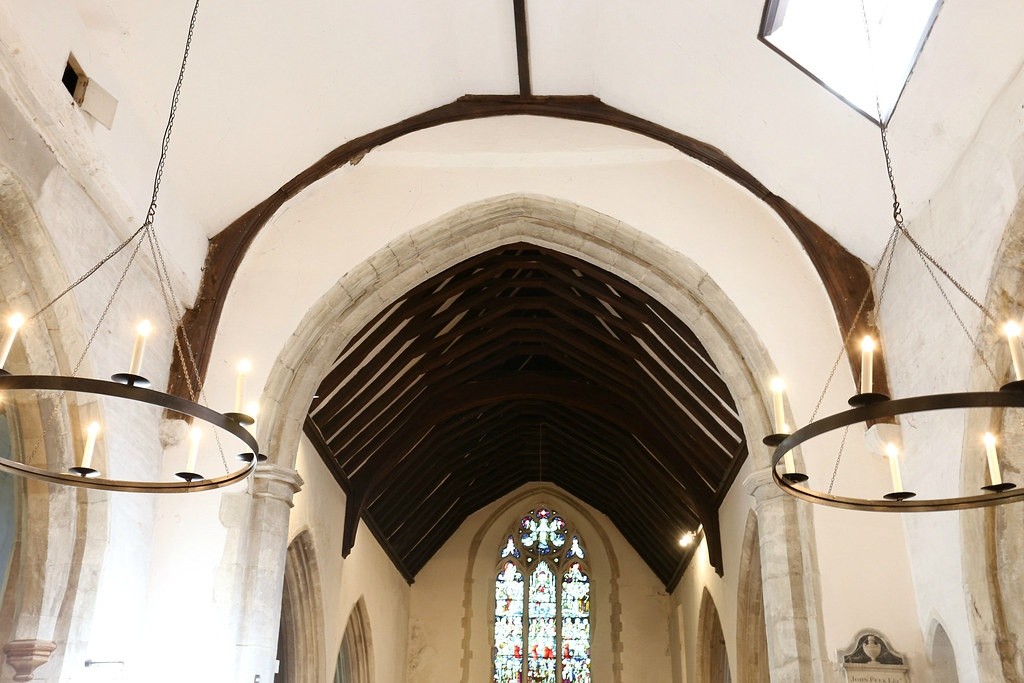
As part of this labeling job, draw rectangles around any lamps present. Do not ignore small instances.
[0,0,264,492]
[762,3,1024,513]
